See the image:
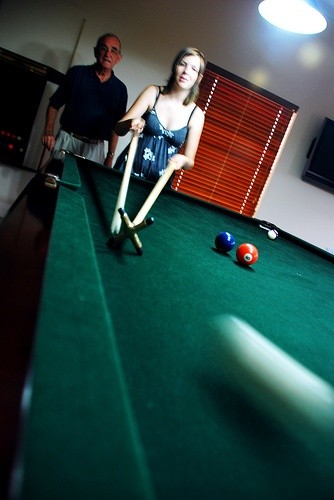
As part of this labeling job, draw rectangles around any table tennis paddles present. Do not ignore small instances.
[267,229,279,240]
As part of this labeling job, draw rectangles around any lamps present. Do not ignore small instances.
[257,0,328,35]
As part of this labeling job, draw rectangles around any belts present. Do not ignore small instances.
[61,127,104,144]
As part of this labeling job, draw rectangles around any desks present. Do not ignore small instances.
[0,151,334,500]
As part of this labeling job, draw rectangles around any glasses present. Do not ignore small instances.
[96,45,120,56]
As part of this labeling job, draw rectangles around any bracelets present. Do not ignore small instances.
[107,151,115,159]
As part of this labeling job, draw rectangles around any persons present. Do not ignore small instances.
[111,46,206,189]
[42,33,127,169]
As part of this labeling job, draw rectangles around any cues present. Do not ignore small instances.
[108,128,139,238]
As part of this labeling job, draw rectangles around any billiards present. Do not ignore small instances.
[236,243,259,266]
[215,231,236,253]
